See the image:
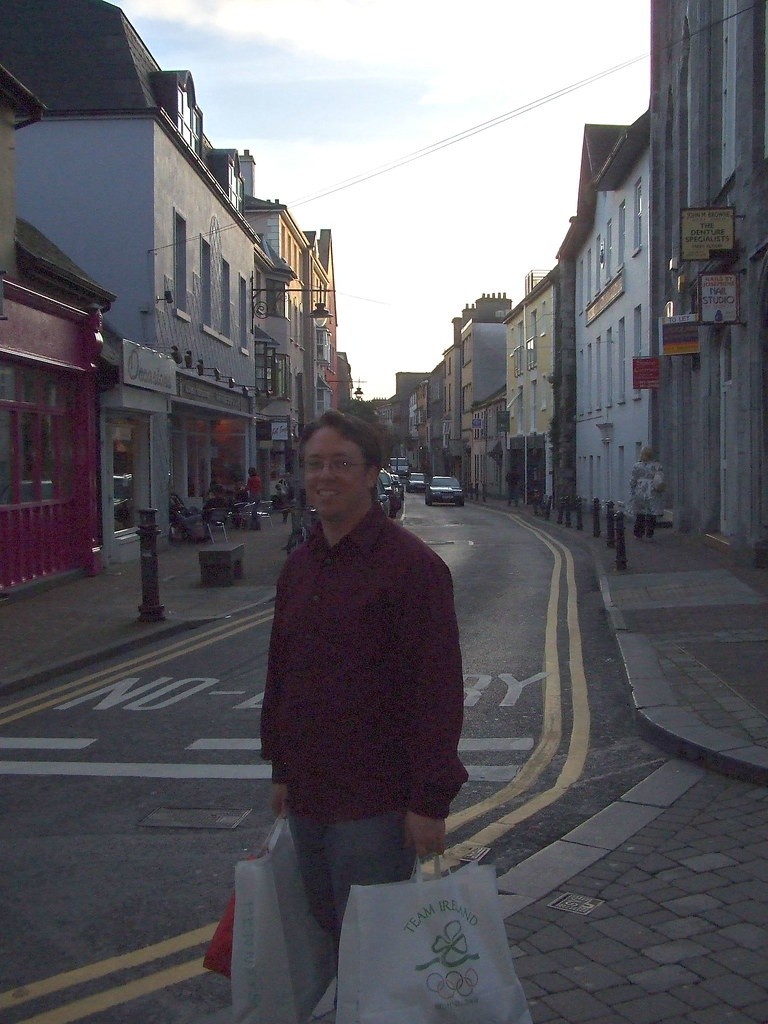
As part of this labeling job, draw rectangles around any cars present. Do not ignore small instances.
[406,473,426,492]
[376,468,404,517]
[425,476,464,506]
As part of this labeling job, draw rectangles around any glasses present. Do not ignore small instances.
[301,457,368,472]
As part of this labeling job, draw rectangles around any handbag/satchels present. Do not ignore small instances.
[337,853,532,1024]
[204,854,256,976]
[234,814,335,1024]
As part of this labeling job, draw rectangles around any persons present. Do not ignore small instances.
[258,408,469,1010]
[168,468,263,543]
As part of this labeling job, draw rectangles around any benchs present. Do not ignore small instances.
[198,542,245,589]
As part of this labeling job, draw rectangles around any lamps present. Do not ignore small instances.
[220,376,236,389]
[326,380,364,396]
[199,368,221,382]
[192,358,204,376]
[251,282,336,334]
[182,350,192,368]
[231,385,250,396]
[244,385,262,398]
[256,390,275,398]
[146,344,183,365]
[157,290,173,304]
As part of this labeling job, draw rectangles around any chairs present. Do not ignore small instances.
[167,494,278,544]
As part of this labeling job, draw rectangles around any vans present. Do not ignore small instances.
[388,457,409,473]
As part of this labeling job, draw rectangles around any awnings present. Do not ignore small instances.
[439,412,453,420]
[316,376,330,389]
[488,435,545,449]
[254,324,280,345]
